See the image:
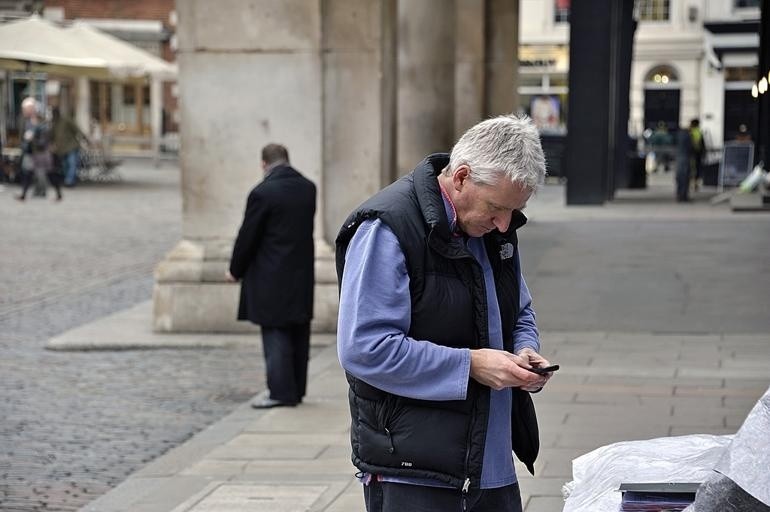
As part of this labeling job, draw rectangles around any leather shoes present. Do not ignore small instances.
[251,399,298,408]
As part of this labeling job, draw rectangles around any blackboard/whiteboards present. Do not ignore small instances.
[718,144,754,187]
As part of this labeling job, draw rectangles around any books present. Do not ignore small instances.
[614,482,702,511]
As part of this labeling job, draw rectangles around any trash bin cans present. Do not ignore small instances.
[626,155,645,189]
[540,135,567,177]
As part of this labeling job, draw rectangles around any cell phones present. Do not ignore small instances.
[533,364,559,374]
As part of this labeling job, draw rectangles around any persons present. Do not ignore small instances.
[716,124,761,178]
[631,118,706,203]
[336,116,554,512]
[222,143,317,409]
[14,97,89,202]
